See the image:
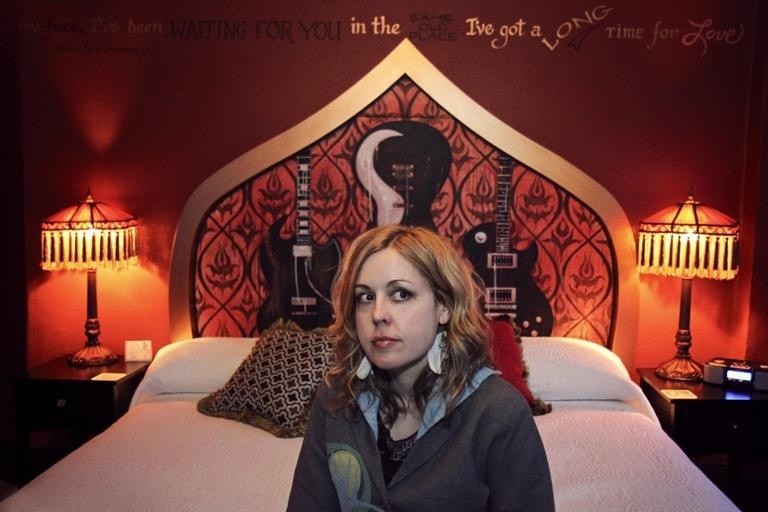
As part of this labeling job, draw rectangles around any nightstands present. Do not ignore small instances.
[12,355,152,489]
[637,364,766,501]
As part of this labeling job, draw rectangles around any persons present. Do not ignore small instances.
[286,219,555,511]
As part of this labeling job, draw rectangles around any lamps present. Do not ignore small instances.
[38,185,143,368]
[634,184,742,381]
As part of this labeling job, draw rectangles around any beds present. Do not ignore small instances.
[4,33,742,511]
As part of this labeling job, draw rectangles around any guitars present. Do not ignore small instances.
[258,149,341,328]
[463,154,554,336]
[350,121,452,233]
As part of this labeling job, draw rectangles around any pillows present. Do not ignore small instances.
[201,315,347,440]
[476,314,554,419]
